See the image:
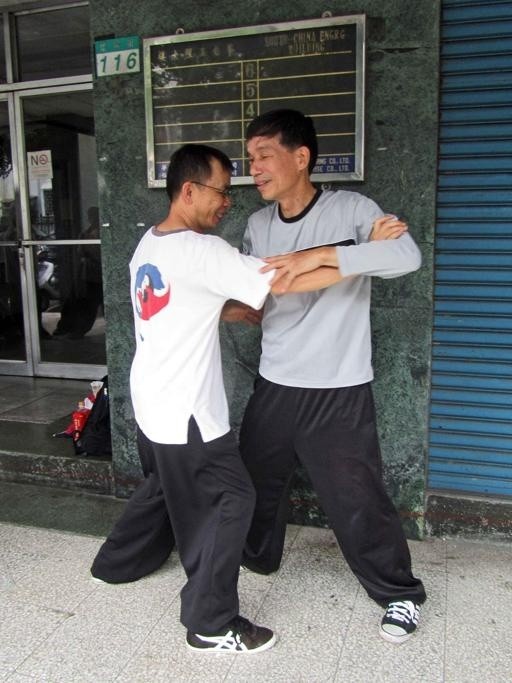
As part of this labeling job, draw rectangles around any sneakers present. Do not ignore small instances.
[185,613,277,655]
[378,598,421,645]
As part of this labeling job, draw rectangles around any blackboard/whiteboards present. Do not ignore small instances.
[142,13,367,190]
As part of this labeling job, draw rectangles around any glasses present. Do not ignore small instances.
[190,181,236,198]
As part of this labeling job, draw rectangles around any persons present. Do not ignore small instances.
[234,110,428,645]
[65,203,100,340]
[91,147,410,650]
